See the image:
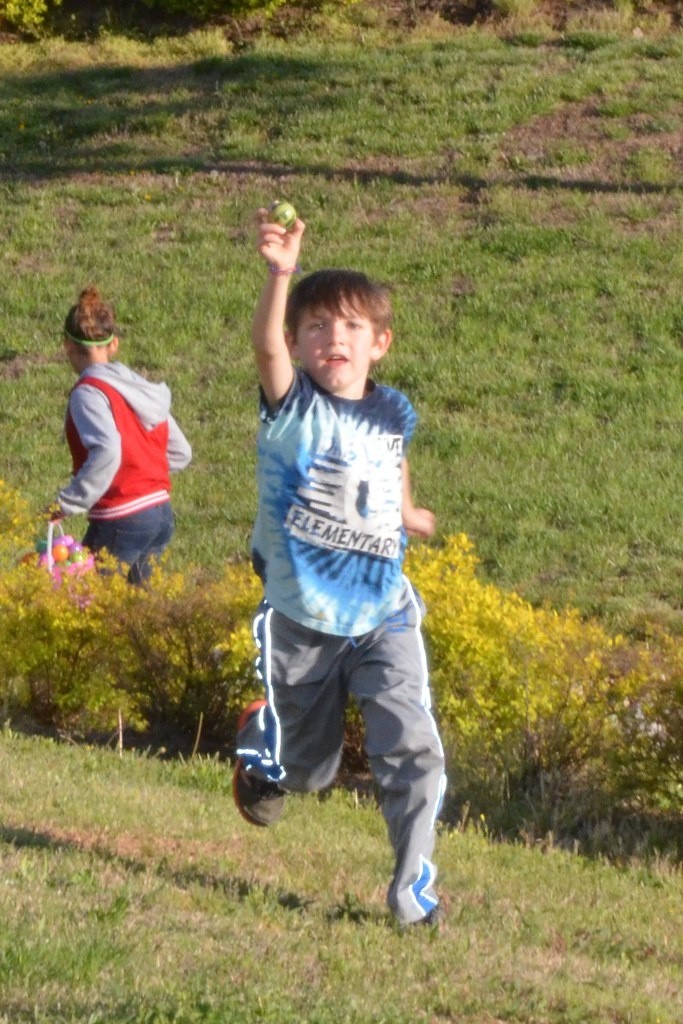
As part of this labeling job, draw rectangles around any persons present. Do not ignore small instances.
[43,286,193,587]
[230,197,448,932]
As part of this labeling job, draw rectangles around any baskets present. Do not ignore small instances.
[17,522,94,608]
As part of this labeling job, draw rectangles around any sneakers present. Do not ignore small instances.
[400,896,439,943]
[232,699,285,827]
